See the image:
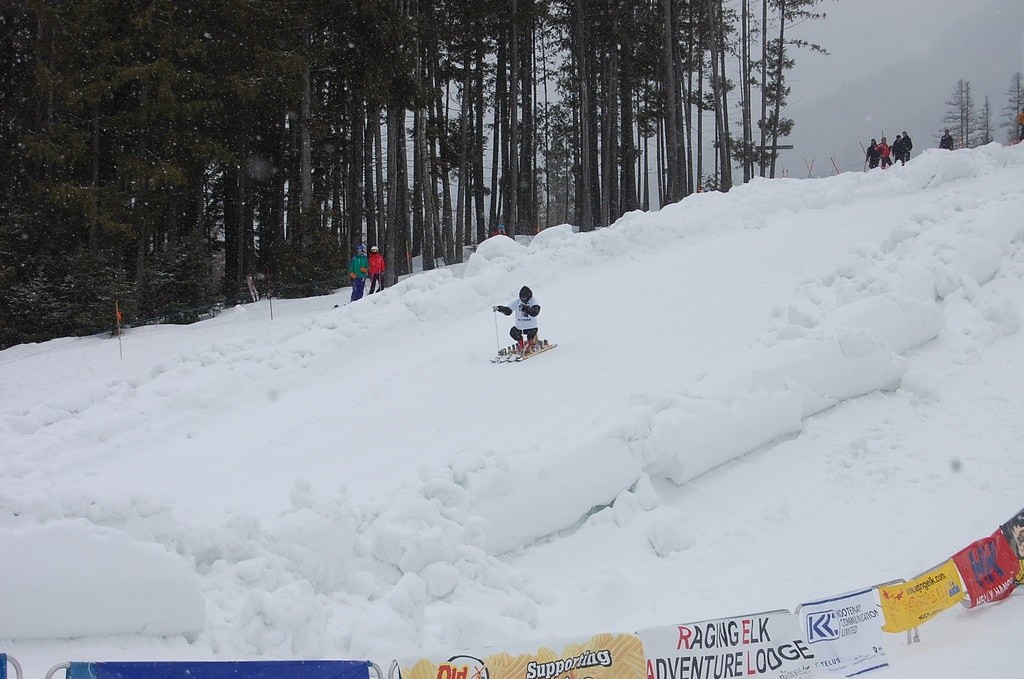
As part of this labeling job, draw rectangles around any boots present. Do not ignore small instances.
[517,339,523,351]
[526,339,534,352]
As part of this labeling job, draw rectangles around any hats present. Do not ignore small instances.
[498,224,504,231]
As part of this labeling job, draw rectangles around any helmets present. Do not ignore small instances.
[357,245,363,250]
[371,246,378,250]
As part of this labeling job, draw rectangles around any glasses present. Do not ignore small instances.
[372,249,377,252]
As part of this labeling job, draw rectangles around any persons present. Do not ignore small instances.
[866,138,881,169]
[492,224,509,237]
[873,137,893,170]
[901,131,913,166]
[892,135,902,163]
[492,286,541,353]
[940,129,953,151]
[1015,109,1024,139]
[348,245,370,302]
[368,246,386,294]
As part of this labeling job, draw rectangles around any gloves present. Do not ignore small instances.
[492,306,499,312]
[381,274,385,280]
[350,273,357,279]
[361,267,367,273]
[521,304,528,311]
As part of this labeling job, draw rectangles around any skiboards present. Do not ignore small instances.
[489,338,557,365]
